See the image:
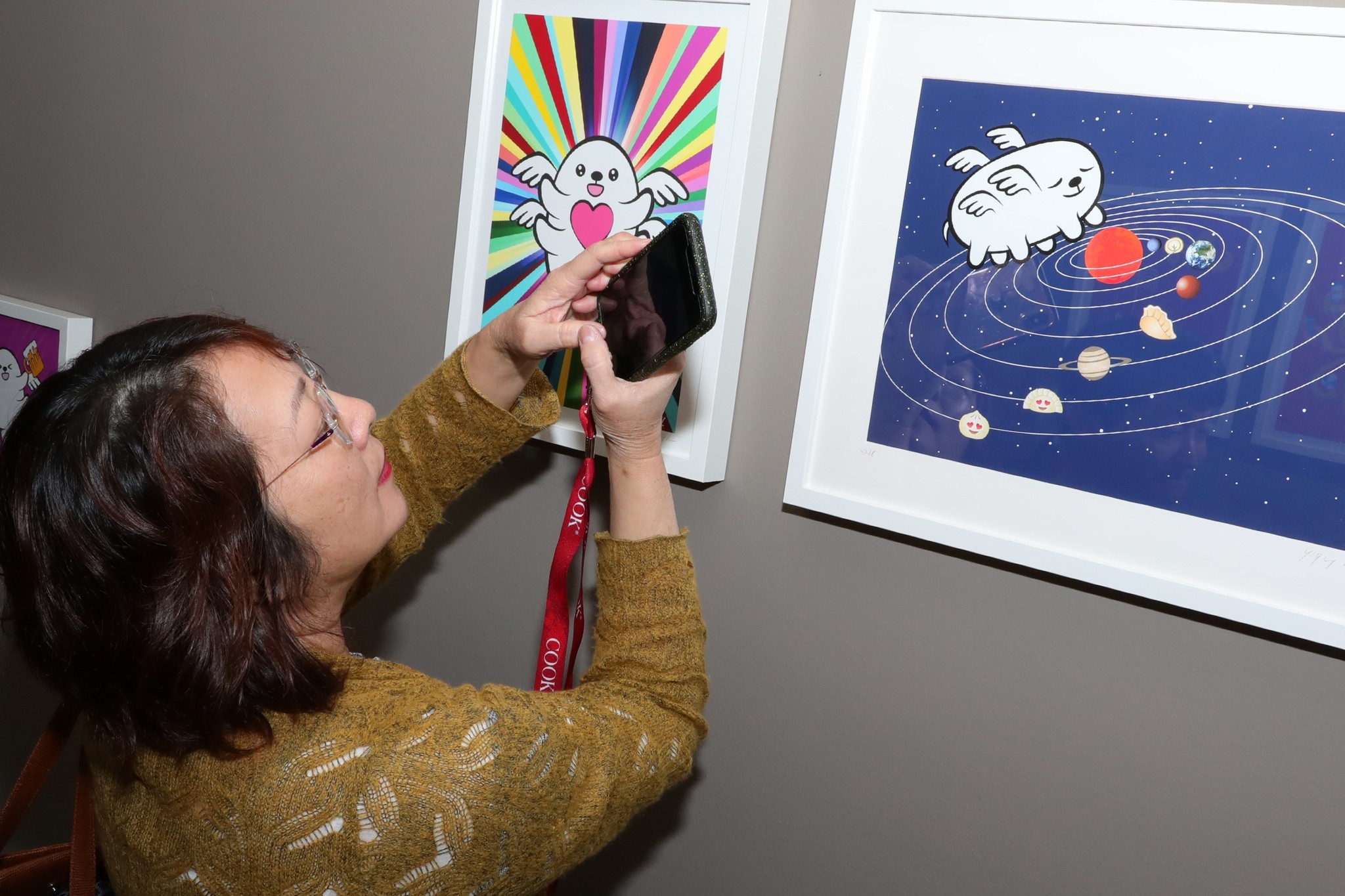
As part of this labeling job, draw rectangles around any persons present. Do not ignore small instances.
[0,231,710,896]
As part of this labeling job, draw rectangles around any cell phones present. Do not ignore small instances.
[597,212,717,383]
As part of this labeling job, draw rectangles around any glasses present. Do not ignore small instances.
[259,339,354,494]
